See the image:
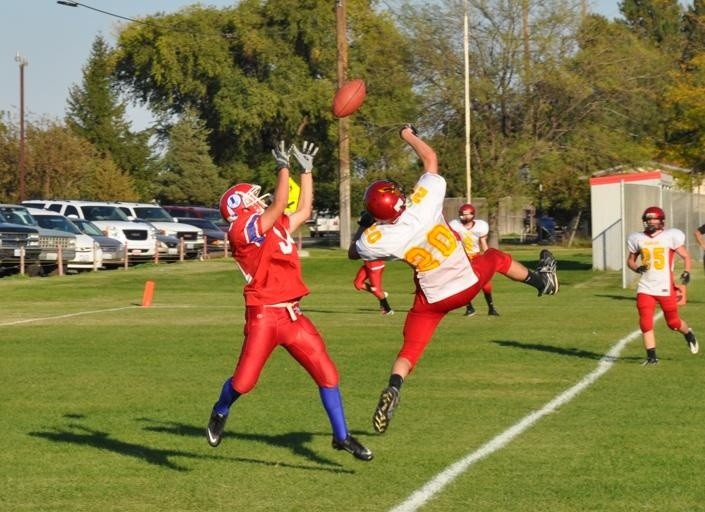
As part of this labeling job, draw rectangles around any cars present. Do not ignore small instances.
[127,218,186,262]
[13,208,103,273]
[0,203,76,272]
[0,210,41,276]
[173,217,231,254]
[66,219,126,270]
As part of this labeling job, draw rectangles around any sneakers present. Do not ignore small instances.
[641,358,659,367]
[381,309,393,316]
[371,386,400,434]
[206,404,228,447]
[535,249,560,297]
[687,328,699,355]
[463,308,476,316]
[488,310,499,316]
[332,434,376,461]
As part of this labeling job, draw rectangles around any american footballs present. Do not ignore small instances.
[333,80,366,117]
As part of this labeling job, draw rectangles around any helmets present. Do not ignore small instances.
[218,182,271,225]
[363,178,408,224]
[641,206,667,229]
[458,203,475,225]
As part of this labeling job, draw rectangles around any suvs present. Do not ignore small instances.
[21,200,157,264]
[310,208,341,238]
[163,206,222,221]
[118,201,204,258]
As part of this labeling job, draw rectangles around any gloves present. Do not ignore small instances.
[636,265,648,275]
[292,142,320,172]
[400,123,418,137]
[358,211,375,226]
[271,139,295,172]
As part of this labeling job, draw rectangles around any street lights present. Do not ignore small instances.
[13,53,33,205]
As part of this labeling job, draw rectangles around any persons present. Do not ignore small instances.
[627,206,699,367]
[353,260,395,317]
[693,224,705,269]
[348,124,560,434]
[206,138,374,462]
[448,204,500,317]
